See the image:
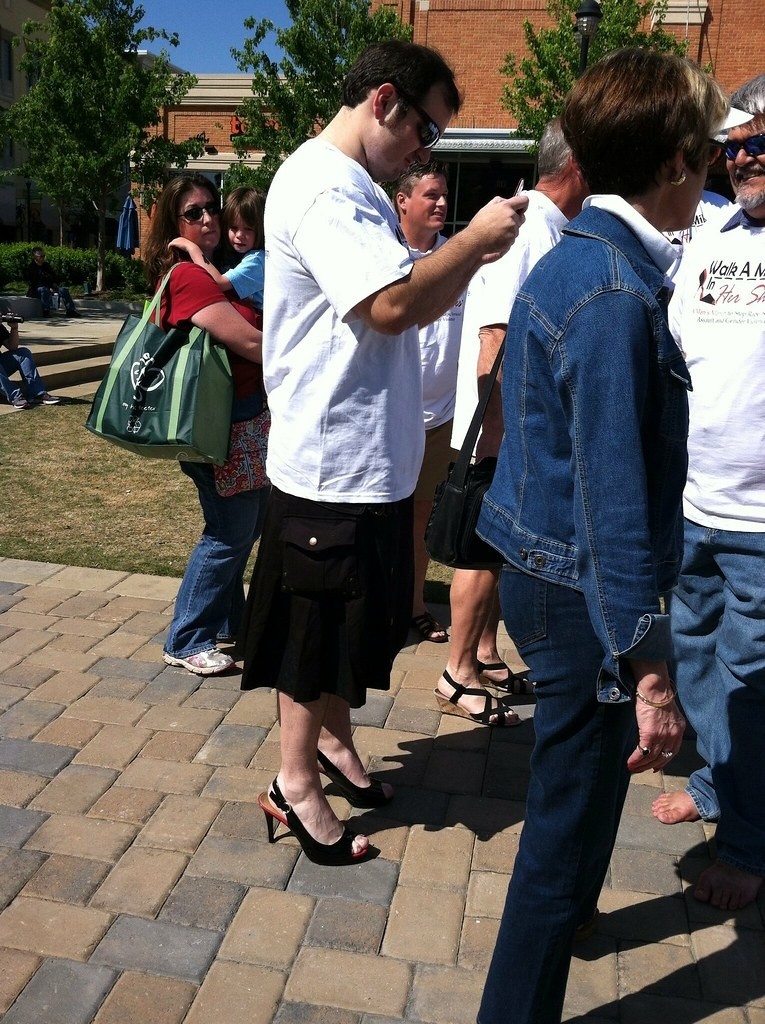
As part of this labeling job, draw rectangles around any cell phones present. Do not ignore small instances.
[512,178,525,196]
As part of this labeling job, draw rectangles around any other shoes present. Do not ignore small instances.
[44,305,53,318]
[66,310,82,318]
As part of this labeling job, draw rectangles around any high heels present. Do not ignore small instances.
[435,669,522,728]
[258,772,368,867]
[316,748,395,809]
[475,657,536,693]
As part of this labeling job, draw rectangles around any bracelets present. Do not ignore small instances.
[634,678,679,706]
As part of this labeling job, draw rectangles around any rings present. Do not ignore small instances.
[662,752,673,757]
[638,744,651,756]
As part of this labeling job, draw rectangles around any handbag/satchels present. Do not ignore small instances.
[422,325,505,570]
[214,407,270,498]
[83,260,232,467]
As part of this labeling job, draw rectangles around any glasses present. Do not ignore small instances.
[35,255,45,257]
[176,202,219,221]
[724,134,765,160]
[703,136,728,166]
[395,85,441,150]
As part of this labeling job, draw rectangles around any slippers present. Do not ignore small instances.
[411,610,448,643]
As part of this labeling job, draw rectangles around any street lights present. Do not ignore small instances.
[576,0,603,73]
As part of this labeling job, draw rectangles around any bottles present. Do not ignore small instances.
[1,314,24,323]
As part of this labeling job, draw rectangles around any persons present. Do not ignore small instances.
[144,174,271,675]
[435,43,764,1024]
[393,156,468,644]
[27,247,80,318]
[0,313,61,408]
[168,187,266,311]
[241,39,530,864]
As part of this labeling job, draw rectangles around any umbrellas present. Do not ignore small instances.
[117,192,140,255]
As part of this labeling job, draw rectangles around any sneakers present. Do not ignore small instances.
[13,395,31,408]
[162,641,243,675]
[28,392,61,405]
